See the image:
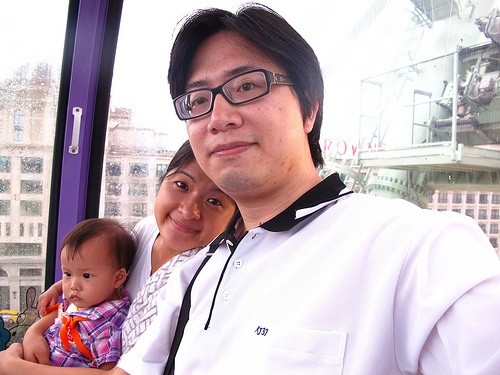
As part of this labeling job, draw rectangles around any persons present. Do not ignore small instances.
[0,137,239,375]
[109,1,500,375]
[35,217,139,371]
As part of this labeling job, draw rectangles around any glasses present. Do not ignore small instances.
[174,68,294,121]
